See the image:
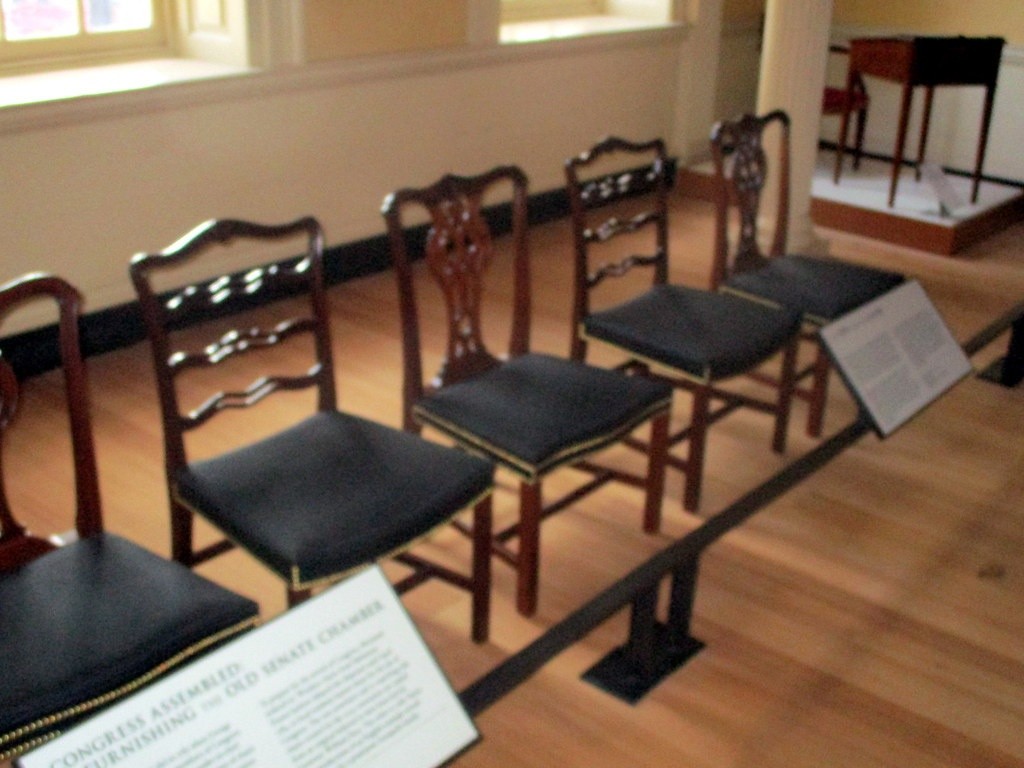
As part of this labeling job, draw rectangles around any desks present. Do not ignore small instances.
[833,35,1008,206]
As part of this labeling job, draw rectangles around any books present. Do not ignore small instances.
[12,562,483,768]
[815,280,975,445]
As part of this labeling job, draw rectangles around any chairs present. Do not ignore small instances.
[132,216,497,642]
[380,163,676,618]
[709,106,907,437]
[0,270,259,764]
[567,133,803,513]
[822,44,872,171]
